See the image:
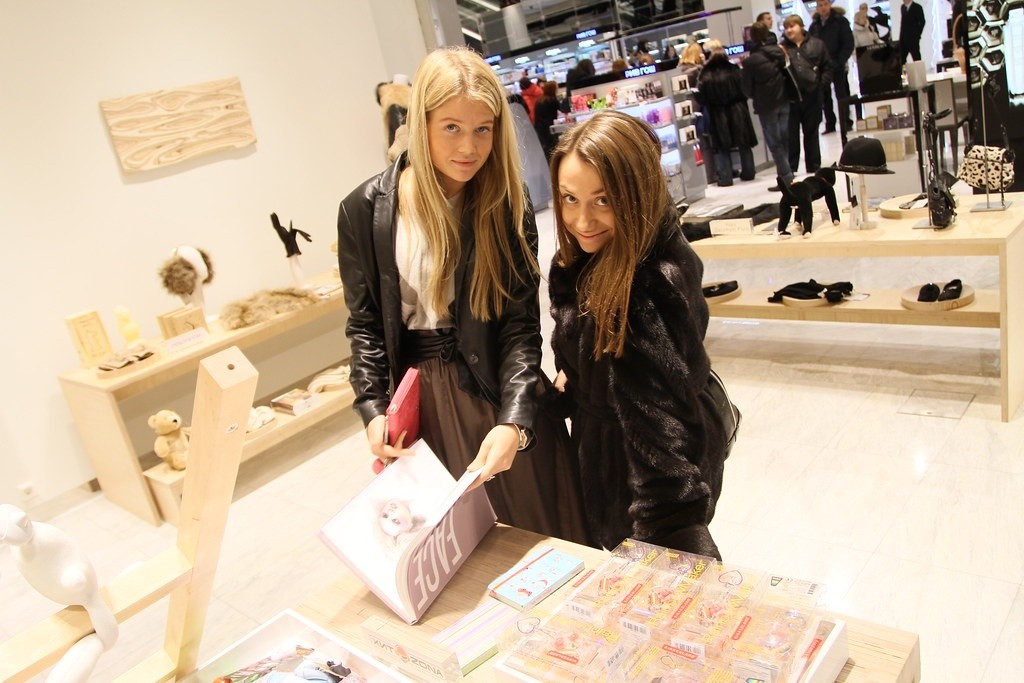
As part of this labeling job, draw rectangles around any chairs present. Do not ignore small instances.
[927,77,970,176]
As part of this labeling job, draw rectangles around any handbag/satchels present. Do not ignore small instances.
[955,144,1015,190]
[928,169,958,230]
[784,66,803,102]
[699,379,743,462]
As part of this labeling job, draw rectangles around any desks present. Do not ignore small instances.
[839,88,926,203]
[183,521,924,683]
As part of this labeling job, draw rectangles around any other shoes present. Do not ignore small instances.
[768,185,781,191]
[846,125,852,132]
[822,129,836,135]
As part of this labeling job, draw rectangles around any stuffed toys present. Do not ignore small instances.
[148,408,188,470]
[246,406,275,433]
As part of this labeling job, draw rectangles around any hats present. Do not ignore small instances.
[830,135,895,175]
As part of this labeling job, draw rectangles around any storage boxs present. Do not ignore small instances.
[855,105,918,162]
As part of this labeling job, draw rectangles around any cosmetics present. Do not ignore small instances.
[558,76,702,168]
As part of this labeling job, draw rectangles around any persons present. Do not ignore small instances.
[498,34,702,155]
[780,15,833,175]
[899,1,925,63]
[742,12,798,191]
[809,0,855,135]
[692,40,755,187]
[549,109,741,563]
[852,0,890,47]
[337,45,583,544]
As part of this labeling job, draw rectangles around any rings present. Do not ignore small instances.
[486,474,496,481]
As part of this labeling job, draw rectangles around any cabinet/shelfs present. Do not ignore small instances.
[550,89,707,206]
[686,212,1024,421]
[56,269,351,525]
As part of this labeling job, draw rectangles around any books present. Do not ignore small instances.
[320,436,499,625]
[271,386,321,415]
